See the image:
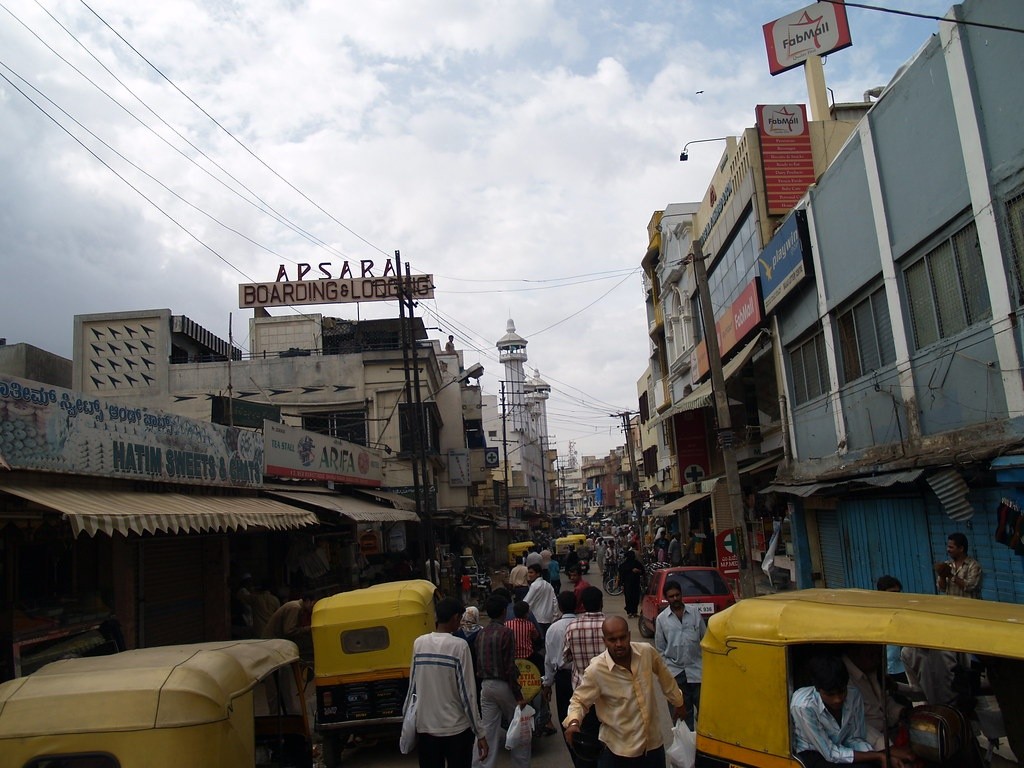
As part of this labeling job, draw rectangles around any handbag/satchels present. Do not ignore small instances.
[399,694,418,755]
[505,703,536,768]
[666,718,697,768]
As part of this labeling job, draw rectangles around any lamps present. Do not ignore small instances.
[360,441,392,455]
[679,135,746,163]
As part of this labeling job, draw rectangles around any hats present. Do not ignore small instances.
[661,531,666,534]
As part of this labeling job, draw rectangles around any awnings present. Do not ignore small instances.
[0,484,421,538]
[652,493,711,517]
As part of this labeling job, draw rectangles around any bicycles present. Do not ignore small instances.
[605,559,624,596]
[601,555,618,593]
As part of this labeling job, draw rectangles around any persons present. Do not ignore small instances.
[472,597,532,768]
[562,586,608,768]
[562,616,685,768]
[790,658,915,768]
[934,532,990,711]
[407,596,489,768]
[231,520,714,732]
[541,591,576,762]
[844,576,1005,768]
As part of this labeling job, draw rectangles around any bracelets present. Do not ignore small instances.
[570,723,577,726]
[951,576,956,581]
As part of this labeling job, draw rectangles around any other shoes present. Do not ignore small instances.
[545,722,557,733]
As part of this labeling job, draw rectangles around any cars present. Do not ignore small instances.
[638,566,736,638]
[458,556,481,575]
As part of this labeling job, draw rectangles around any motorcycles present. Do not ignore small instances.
[0,636,316,768]
[467,569,493,613]
[693,588,1023,768]
[312,579,450,768]
[508,542,538,571]
[554,534,591,587]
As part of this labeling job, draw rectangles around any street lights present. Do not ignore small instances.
[505,438,539,543]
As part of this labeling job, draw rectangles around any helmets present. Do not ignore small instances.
[571,731,606,761]
